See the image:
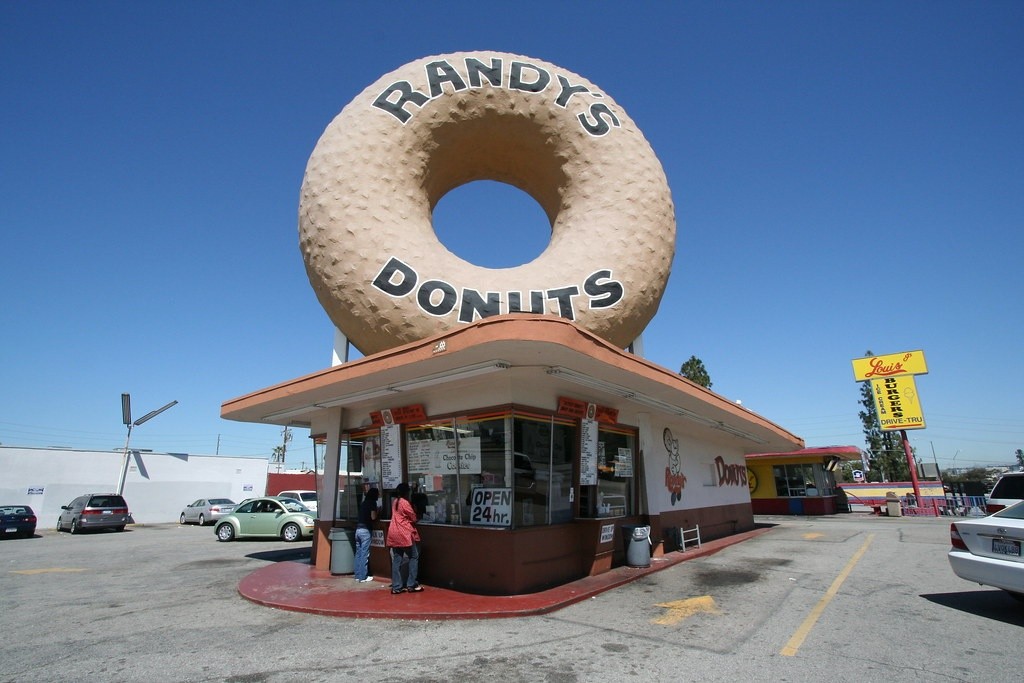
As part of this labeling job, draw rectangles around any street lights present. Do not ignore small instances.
[116,392,179,495]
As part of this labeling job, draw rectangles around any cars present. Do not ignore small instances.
[274,489,317,513]
[56,492,129,535]
[0,505,37,540]
[986,472,1024,517]
[214,495,317,542]
[179,498,238,526]
[948,500,1024,594]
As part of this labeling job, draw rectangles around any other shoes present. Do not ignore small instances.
[391,589,406,594]
[407,586,425,593]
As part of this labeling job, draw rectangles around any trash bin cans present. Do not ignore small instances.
[885,491,902,517]
[621,524,651,568]
[328,526,356,574]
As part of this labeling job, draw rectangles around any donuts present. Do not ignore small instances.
[299,48,675,350]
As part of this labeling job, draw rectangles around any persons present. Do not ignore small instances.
[409,484,428,519]
[353,487,380,585]
[904,492,918,514]
[466,474,486,506]
[386,482,424,594]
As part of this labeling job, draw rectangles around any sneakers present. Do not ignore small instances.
[355,576,374,583]
